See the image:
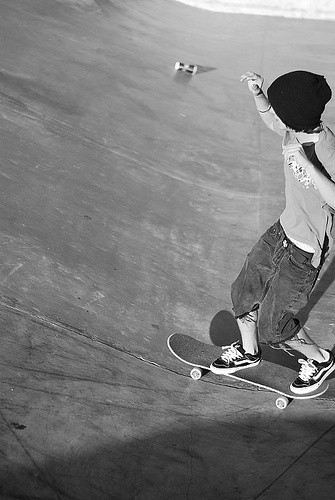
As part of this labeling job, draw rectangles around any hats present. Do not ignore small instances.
[267,71,331,129]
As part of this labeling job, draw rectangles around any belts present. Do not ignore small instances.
[275,219,315,265]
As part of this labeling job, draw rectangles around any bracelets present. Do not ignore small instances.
[257,103,271,113]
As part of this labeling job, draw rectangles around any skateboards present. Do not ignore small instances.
[166,332,329,409]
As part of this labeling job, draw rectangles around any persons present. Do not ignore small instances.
[211,71,335,395]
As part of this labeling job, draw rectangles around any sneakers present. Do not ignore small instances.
[211,345,262,375]
[290,350,334,394]
